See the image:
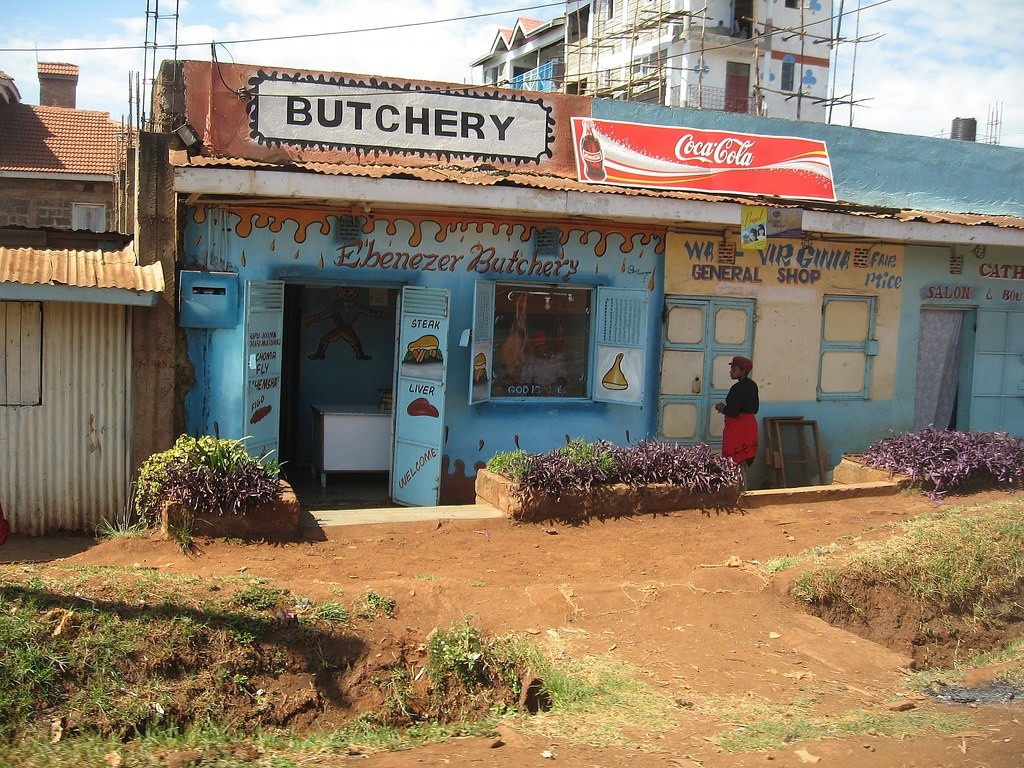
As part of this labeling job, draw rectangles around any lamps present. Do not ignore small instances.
[177,125,201,153]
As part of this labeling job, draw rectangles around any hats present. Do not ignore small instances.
[728,356,753,372]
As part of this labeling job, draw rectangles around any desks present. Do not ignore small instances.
[310,405,392,486]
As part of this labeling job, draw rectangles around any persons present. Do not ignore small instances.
[715,357,759,468]
[750,224,766,242]
